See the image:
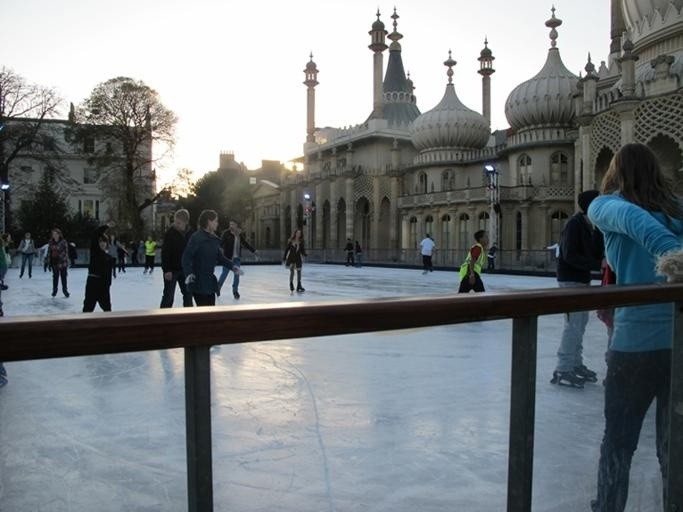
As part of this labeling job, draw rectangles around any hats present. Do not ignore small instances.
[577,190,599,213]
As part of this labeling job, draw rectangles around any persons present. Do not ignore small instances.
[181,210,241,306]
[217,219,257,299]
[16,232,37,278]
[586,143,683,511]
[355,241,362,267]
[108,234,161,278]
[68,240,77,268]
[550,190,608,389]
[1,233,13,387]
[458,230,489,293]
[486,242,498,273]
[46,228,70,298]
[82,220,115,312]
[344,238,355,266]
[39,239,52,272]
[544,242,560,258]
[159,210,193,308]
[283,228,307,292]
[419,233,435,274]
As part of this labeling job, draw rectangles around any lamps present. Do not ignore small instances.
[304,192,310,201]
[484,162,499,175]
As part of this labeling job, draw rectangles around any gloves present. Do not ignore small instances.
[185,274,197,286]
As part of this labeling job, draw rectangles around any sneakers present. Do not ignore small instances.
[560,371,586,384]
[574,366,596,378]
[297,284,304,290]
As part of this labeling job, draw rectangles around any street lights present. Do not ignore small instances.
[0,184,9,234]
[303,193,311,259]
[484,164,495,266]
